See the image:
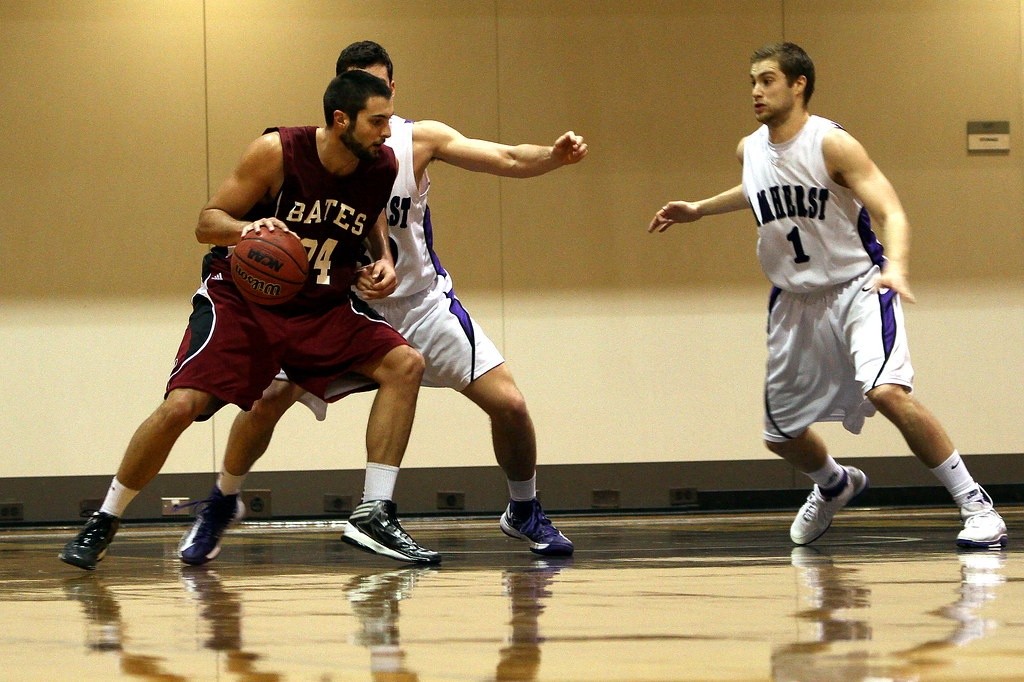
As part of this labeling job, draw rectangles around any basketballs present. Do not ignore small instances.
[231,226,309,304]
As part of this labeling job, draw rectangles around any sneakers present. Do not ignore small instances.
[500,503,574,557]
[172,485,246,566]
[58,509,122,571]
[789,465,867,547]
[956,484,1008,547]
[340,500,441,566]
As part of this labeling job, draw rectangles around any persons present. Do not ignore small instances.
[772,547,1003,682]
[647,42,1005,545]
[178,42,589,566]
[58,70,441,571]
[61,571,563,682]
